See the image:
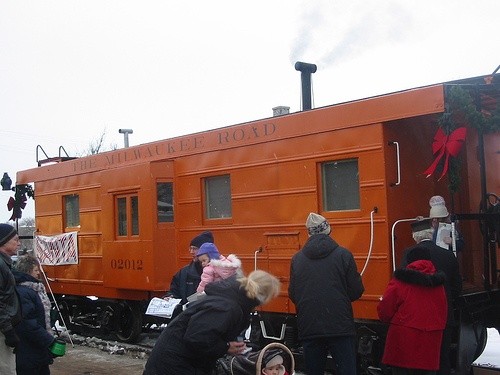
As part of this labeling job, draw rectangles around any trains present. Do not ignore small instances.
[0,77,499,367]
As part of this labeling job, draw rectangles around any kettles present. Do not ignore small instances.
[48,337,67,357]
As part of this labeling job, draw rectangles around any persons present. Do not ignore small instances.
[163,231,215,320]
[377,246,448,375]
[141,270,280,375]
[404,219,463,375]
[13,255,65,375]
[196,243,241,296]
[429,195,465,254]
[0,223,21,375]
[262,355,288,375]
[288,211,365,375]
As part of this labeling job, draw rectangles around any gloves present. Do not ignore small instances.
[5,329,19,347]
[50,309,59,321]
[47,335,54,347]
[443,237,451,245]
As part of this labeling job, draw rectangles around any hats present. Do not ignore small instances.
[407,246,431,263]
[190,231,214,248]
[196,243,219,260]
[429,196,449,218]
[266,355,283,368]
[410,219,432,232]
[0,224,18,246]
[305,212,331,237]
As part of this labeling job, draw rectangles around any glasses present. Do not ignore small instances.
[188,247,199,251]
